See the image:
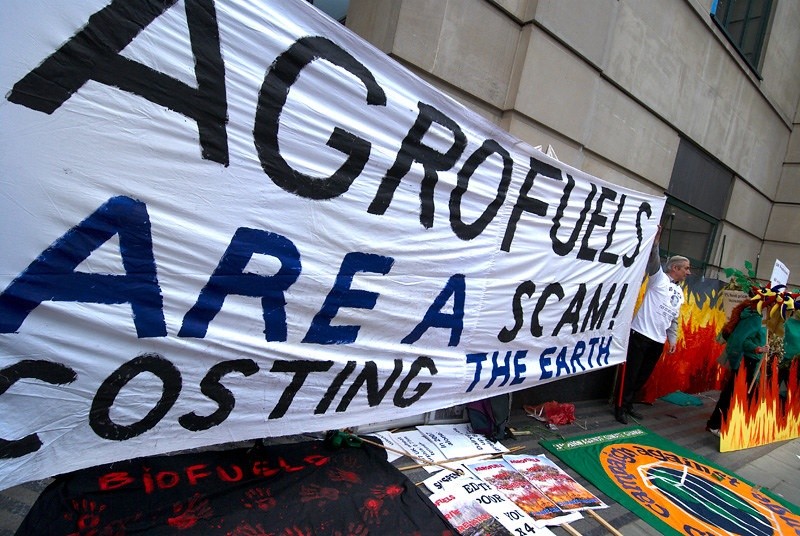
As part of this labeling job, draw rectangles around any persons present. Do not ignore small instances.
[612,225,691,425]
[705,280,800,438]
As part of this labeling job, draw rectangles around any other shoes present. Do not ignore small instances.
[707,428,721,438]
[628,406,644,420]
[614,406,628,425]
